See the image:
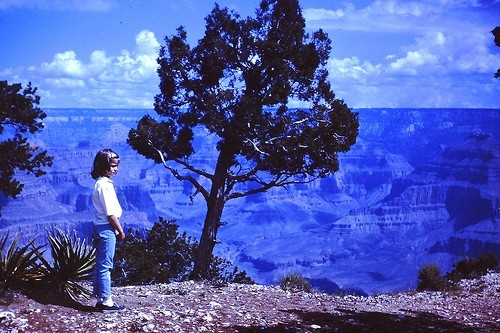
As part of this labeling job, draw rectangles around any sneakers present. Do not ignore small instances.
[95,301,103,309]
[101,302,126,312]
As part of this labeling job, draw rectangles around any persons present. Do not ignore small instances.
[90,149,127,313]
